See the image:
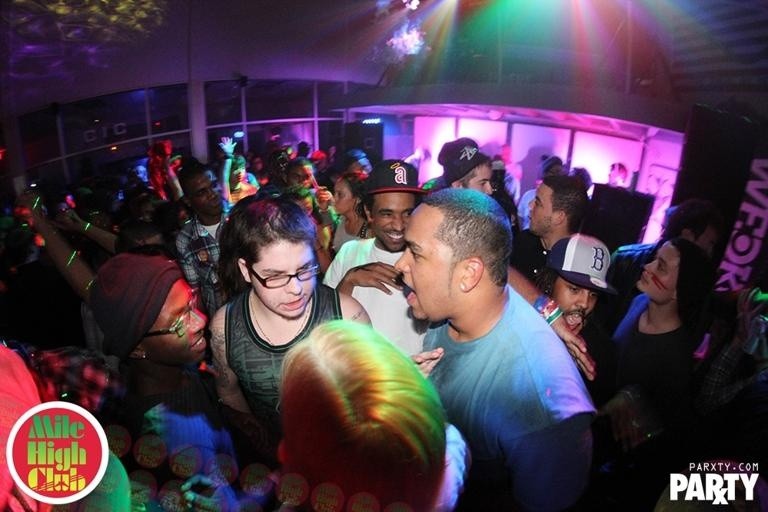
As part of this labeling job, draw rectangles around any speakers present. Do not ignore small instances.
[581,184,656,250]
[344,121,383,165]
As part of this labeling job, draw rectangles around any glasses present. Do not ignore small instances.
[245,259,322,289]
[143,286,201,339]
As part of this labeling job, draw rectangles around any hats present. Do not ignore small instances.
[540,153,563,171]
[545,234,618,294]
[363,158,429,196]
[87,251,186,357]
[334,147,366,174]
[439,137,489,185]
